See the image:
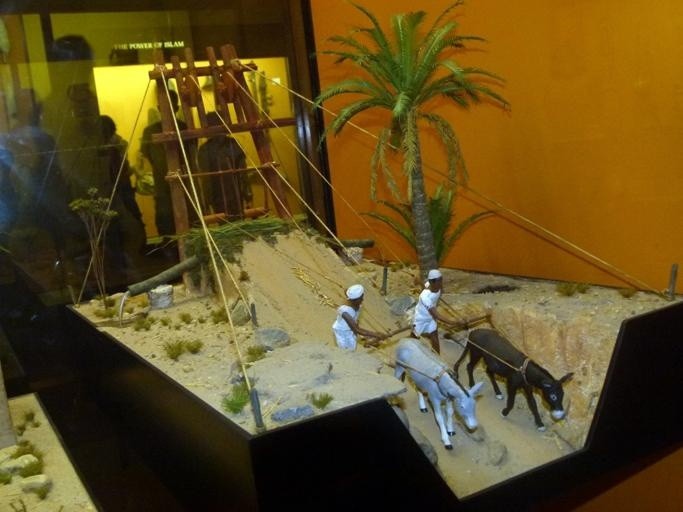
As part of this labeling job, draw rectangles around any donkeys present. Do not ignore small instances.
[454,328,576,431]
[394,336,485,451]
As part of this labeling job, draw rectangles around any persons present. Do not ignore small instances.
[409,268,468,355]
[64,87,150,262]
[0,86,70,239]
[194,112,252,224]
[332,285,391,350]
[37,35,144,300]
[138,91,198,239]
[82,112,152,280]
